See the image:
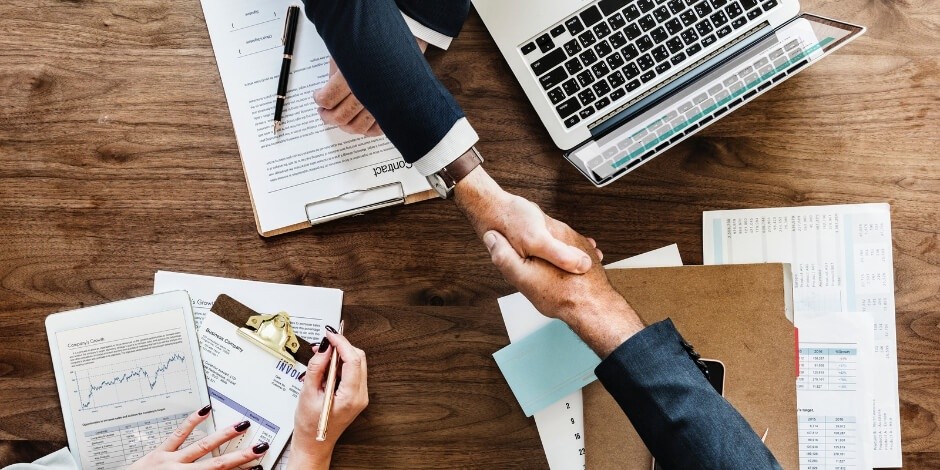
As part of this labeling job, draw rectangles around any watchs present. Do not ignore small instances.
[425,145,484,200]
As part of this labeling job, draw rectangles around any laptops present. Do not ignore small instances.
[471,0,867,187]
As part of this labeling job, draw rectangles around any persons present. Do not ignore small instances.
[123,325,370,470]
[483,202,785,470]
[301,1,603,274]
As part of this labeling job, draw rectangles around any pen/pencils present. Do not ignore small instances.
[274,3,300,135]
[315,319,345,441]
